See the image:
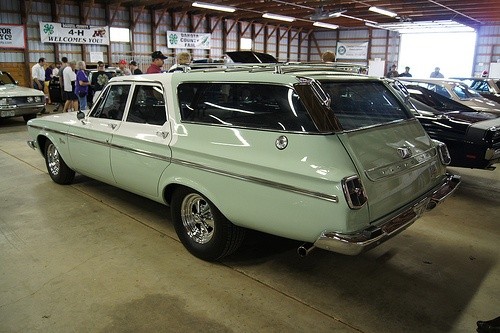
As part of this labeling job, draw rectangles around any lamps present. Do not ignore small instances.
[262,13,295,23]
[191,1,236,13]
[312,22,339,29]
[369,5,397,17]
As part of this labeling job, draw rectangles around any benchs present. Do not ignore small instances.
[225,111,315,132]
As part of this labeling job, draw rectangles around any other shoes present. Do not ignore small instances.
[41,111,50,114]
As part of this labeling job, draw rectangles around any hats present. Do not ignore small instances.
[152,50,168,59]
[120,60,126,64]
[129,60,137,65]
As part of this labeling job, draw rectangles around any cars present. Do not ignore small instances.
[321,77,500,195]
[0,69,46,123]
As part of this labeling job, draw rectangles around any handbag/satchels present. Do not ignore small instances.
[78,91,87,98]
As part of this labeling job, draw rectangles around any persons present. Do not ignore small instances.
[321,50,335,63]
[109,95,127,120]
[32,57,144,114]
[144,51,168,101]
[397,66,412,77]
[168,52,192,73]
[429,67,444,91]
[386,64,400,78]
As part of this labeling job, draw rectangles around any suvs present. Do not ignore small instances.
[27,50,463,263]
